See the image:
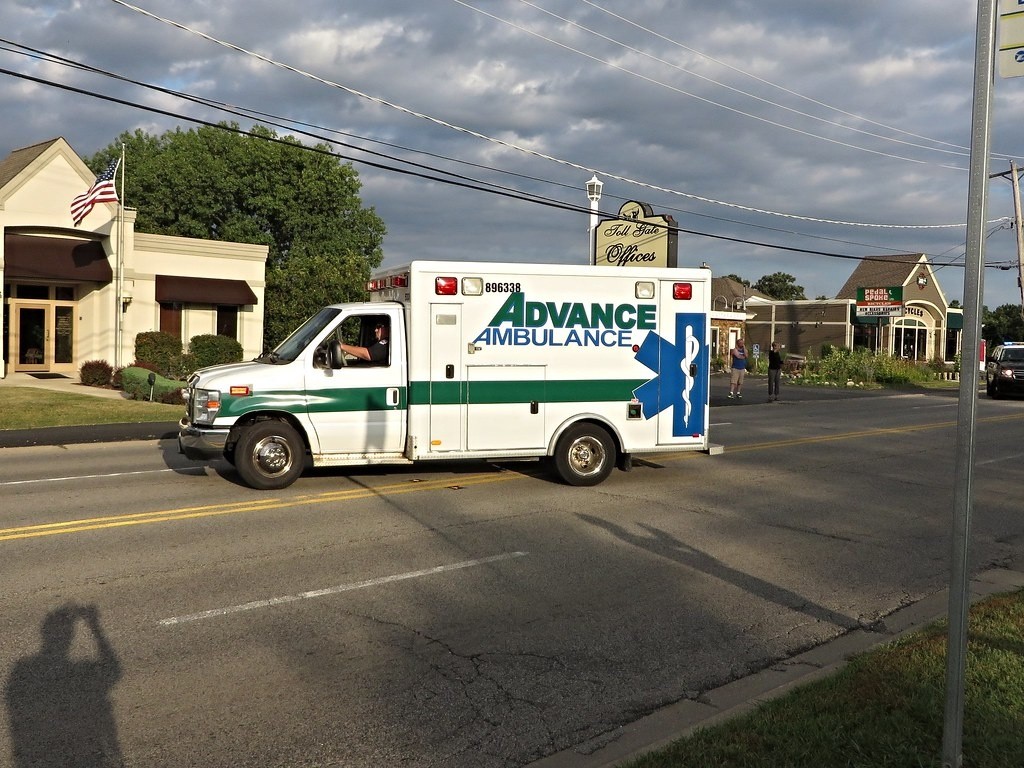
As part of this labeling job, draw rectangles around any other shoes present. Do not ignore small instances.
[768,396,772,402]
[736,394,742,399]
[774,398,780,401]
[727,393,733,399]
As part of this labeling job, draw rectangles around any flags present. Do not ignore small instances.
[70,156,121,228]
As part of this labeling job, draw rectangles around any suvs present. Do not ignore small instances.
[984,342,1023,398]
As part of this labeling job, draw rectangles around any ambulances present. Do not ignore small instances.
[177,261,713,494]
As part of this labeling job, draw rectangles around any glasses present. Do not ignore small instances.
[375,324,384,328]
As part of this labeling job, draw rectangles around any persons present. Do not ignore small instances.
[727,339,748,399]
[767,342,785,401]
[266,281,389,367]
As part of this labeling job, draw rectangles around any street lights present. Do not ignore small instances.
[584,174,604,266]
